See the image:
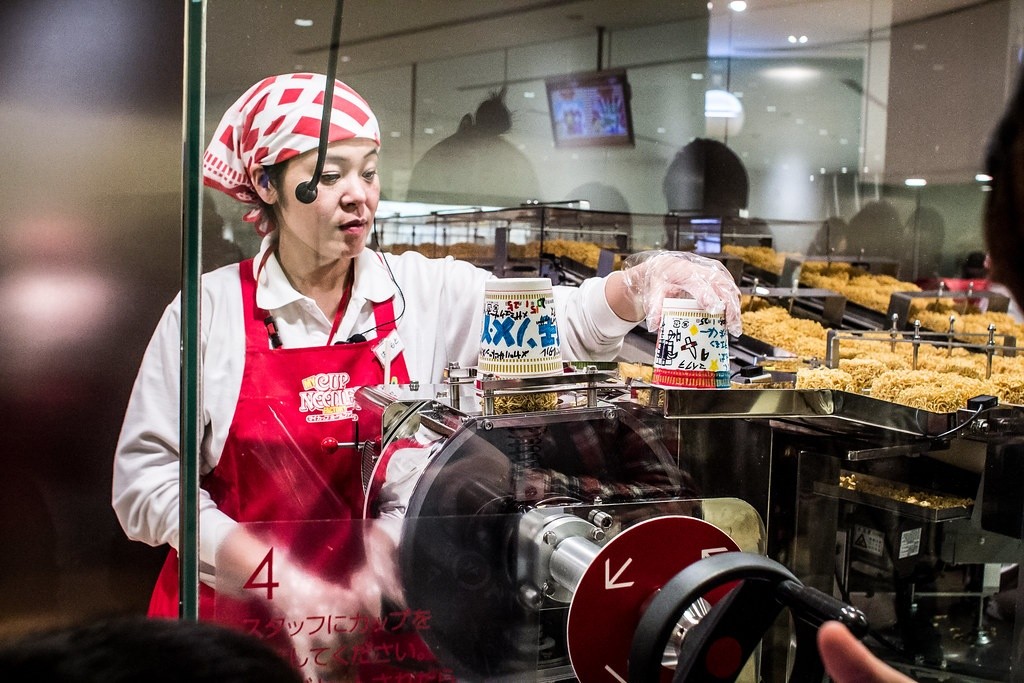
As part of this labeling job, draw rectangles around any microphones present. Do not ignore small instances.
[347,334,367,344]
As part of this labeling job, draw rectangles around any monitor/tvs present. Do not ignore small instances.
[545,69,635,150]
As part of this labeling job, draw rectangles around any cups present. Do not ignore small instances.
[649,295,733,389]
[474,276,565,391]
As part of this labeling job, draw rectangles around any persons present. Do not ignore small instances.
[111,73,743,683]
